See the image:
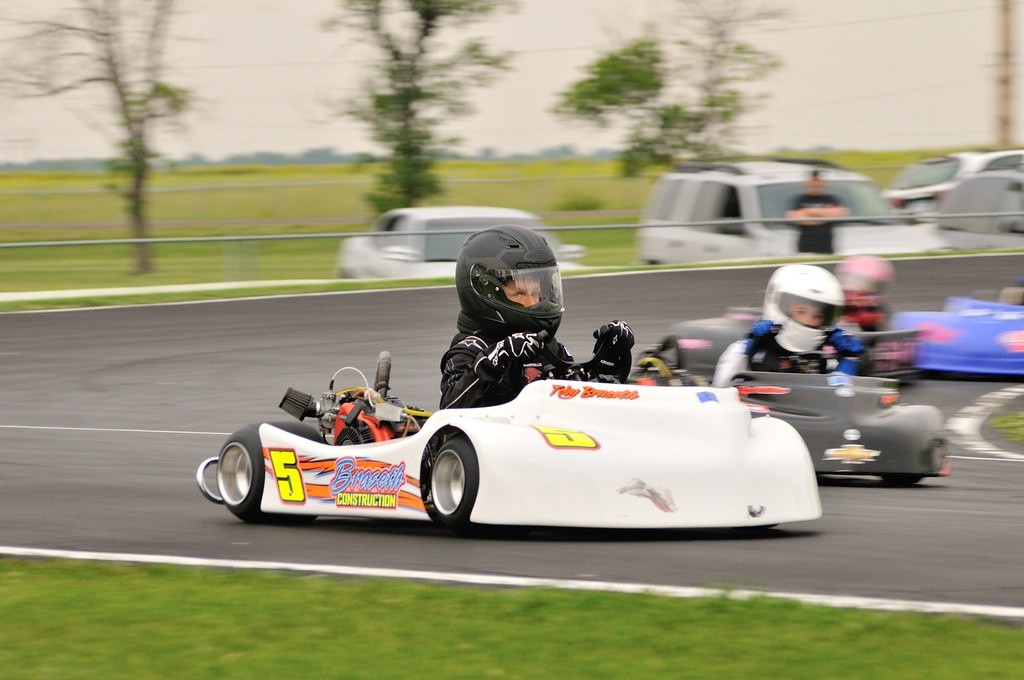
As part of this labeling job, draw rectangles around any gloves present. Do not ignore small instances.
[743,319,782,354]
[473,331,545,386]
[593,320,635,372]
[825,328,864,373]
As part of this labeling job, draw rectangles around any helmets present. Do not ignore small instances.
[762,265,845,355]
[456,224,562,336]
[838,256,894,308]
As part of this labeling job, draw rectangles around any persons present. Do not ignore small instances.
[835,253,894,332]
[712,263,865,391]
[787,170,847,254]
[439,224,634,408]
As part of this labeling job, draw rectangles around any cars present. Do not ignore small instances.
[336,206,587,278]
[938,163,1023,250]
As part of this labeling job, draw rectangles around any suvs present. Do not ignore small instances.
[880,148,1024,211]
[635,156,949,266]
